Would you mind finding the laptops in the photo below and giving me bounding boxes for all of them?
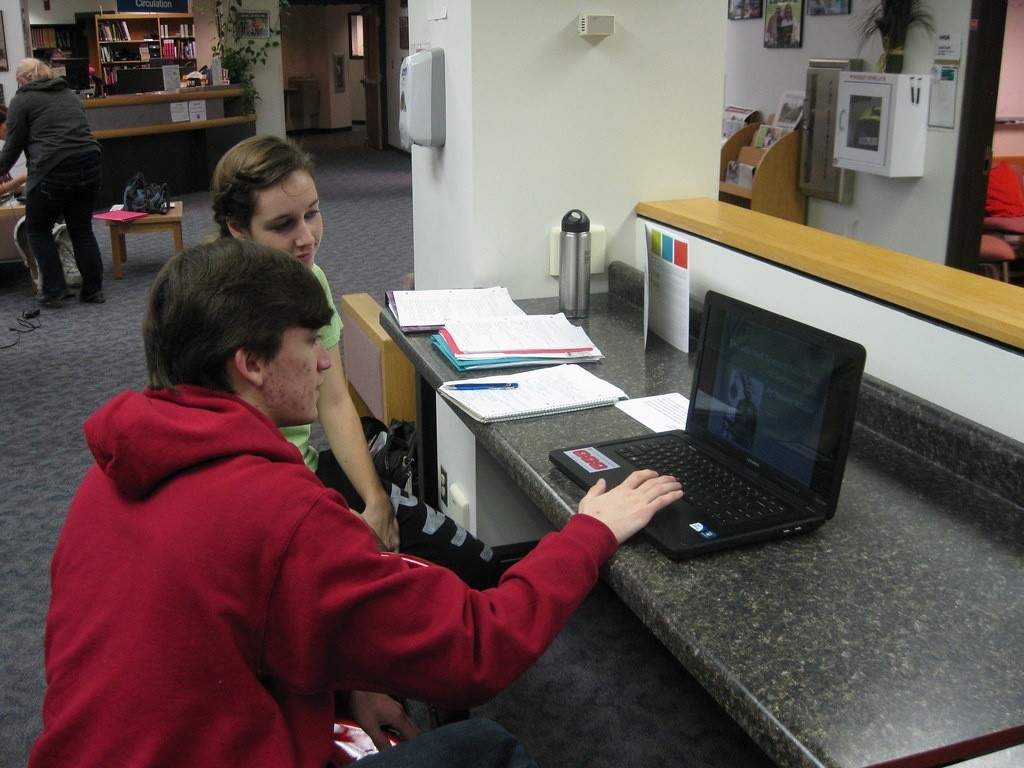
[550,289,867,562]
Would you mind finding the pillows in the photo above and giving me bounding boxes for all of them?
[985,163,1024,218]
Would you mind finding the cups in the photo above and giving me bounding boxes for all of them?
[558,209,589,318]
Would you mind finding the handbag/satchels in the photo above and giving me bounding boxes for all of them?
[124,174,169,215]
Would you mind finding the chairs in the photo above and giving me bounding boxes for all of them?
[978,232,1016,283]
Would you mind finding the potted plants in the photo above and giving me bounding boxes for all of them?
[194,0,291,117]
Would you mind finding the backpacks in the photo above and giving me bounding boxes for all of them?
[360,416,418,493]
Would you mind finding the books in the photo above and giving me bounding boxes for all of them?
[384,286,630,424]
[101,46,151,85]
[160,25,196,59]
[98,20,132,41]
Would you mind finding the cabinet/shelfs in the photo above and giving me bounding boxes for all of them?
[74,11,195,97]
[719,117,805,226]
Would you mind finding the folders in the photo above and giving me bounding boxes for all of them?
[93,211,148,223]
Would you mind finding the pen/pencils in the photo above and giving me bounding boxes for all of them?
[441,383,518,391]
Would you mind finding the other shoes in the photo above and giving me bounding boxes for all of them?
[78,289,105,303]
[36,282,68,302]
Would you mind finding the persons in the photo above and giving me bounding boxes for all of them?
[26,238,683,768]
[45,49,65,69]
[0,57,106,306]
[0,104,28,197]
[210,133,501,729]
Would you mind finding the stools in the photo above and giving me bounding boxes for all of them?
[105,201,183,280]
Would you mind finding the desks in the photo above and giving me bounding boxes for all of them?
[379,312,1024,768]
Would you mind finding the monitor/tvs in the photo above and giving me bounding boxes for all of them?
[114,68,166,94]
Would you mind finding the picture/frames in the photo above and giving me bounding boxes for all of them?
[233,10,269,41]
[728,0,760,19]
[807,0,851,16]
[763,0,803,50]
